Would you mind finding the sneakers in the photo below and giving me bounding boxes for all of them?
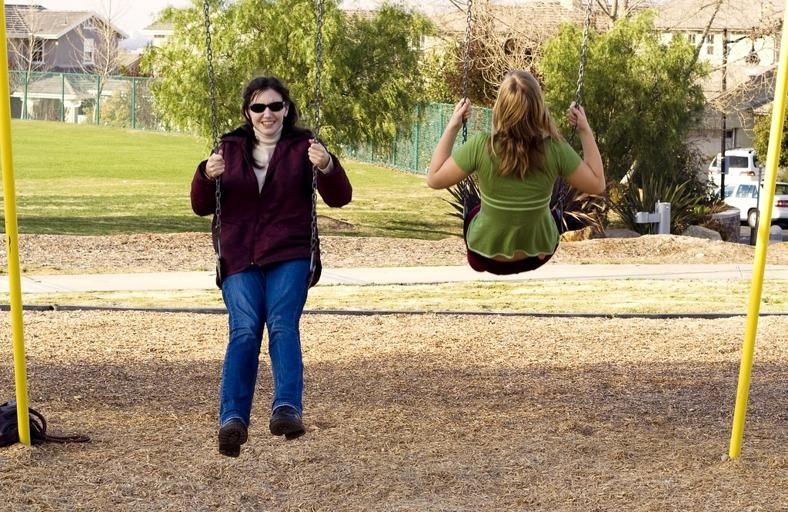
[269,406,306,440]
[218,421,247,457]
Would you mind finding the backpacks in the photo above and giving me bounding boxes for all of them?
[0,400,47,446]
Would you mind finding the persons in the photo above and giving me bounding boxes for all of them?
[427,68,606,275]
[191,78,353,458]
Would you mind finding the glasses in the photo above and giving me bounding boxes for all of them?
[249,102,283,113]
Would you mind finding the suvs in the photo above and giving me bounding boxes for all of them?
[709,181,788,230]
[708,145,785,194]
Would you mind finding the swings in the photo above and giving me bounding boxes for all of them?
[462,1,593,274]
[204,1,323,288]
